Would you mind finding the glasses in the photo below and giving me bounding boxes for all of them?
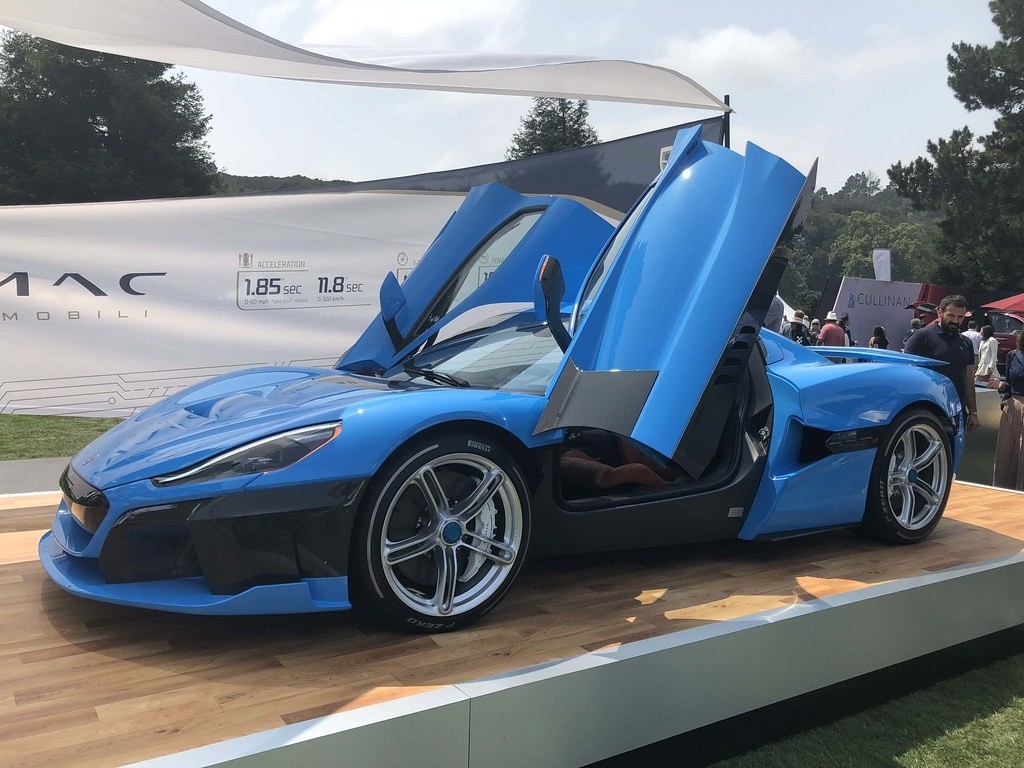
[812,323,819,325]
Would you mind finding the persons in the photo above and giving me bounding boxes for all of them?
[763,294,785,334]
[987,327,1024,491]
[961,319,1001,382]
[869,325,891,349]
[902,313,926,348]
[904,294,980,431]
[782,310,858,364]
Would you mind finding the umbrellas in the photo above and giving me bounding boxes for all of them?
[979,293,1024,324]
[904,298,938,314]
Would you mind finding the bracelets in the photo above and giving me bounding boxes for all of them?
[969,412,977,416]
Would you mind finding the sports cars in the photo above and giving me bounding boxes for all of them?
[38,123,965,634]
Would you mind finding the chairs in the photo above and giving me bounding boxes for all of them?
[562,435,681,495]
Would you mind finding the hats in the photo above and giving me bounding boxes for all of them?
[824,311,838,320]
